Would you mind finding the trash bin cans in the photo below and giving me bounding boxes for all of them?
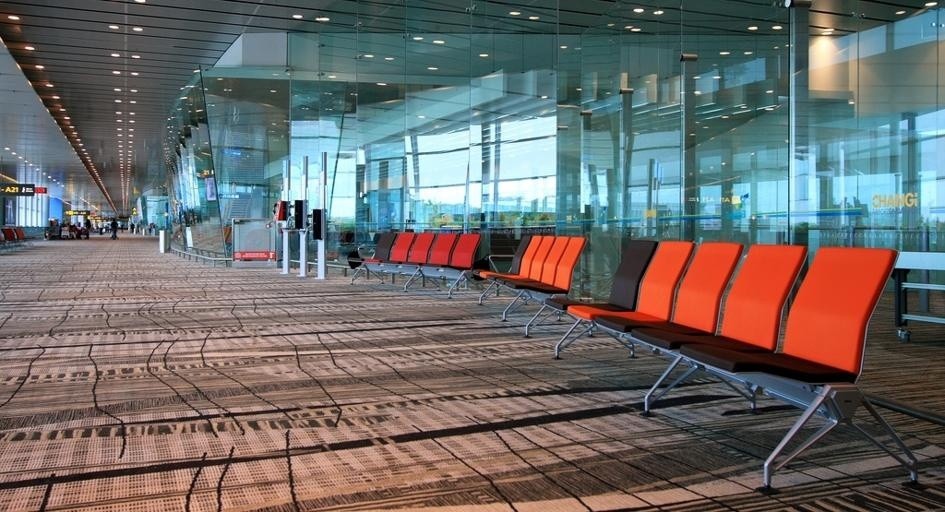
[159,230,170,253]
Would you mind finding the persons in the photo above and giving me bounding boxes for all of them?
[83,219,91,240]
[97,220,104,235]
[130,222,134,233]
[104,223,109,233]
[69,225,82,240]
[110,218,118,240]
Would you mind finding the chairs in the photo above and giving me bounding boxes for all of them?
[347,228,482,299]
